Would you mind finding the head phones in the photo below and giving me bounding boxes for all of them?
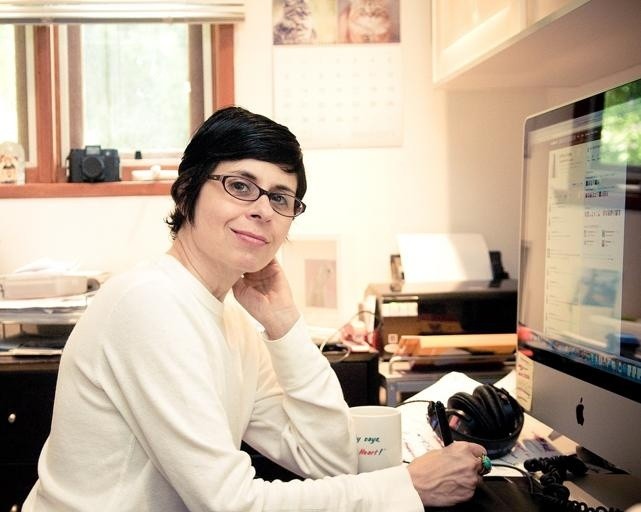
[428,381,526,457]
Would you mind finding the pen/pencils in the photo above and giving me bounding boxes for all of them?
[437,401,453,448]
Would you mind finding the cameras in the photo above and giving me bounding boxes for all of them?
[69,145,120,181]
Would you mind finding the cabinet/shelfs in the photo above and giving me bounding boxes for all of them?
[429,1,641,89]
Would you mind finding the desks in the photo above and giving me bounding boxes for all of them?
[377,361,516,407]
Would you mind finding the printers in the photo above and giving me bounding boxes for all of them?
[362,226,522,368]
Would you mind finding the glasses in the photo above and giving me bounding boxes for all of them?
[203,172,305,217]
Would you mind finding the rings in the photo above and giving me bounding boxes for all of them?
[478,454,492,476]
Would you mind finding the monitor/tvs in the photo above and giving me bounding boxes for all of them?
[515,76,641,512]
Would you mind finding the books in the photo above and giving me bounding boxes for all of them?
[0,262,113,361]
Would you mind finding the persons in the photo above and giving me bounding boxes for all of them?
[22,107,492,511]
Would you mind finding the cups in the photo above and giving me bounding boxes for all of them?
[349,405,402,474]
